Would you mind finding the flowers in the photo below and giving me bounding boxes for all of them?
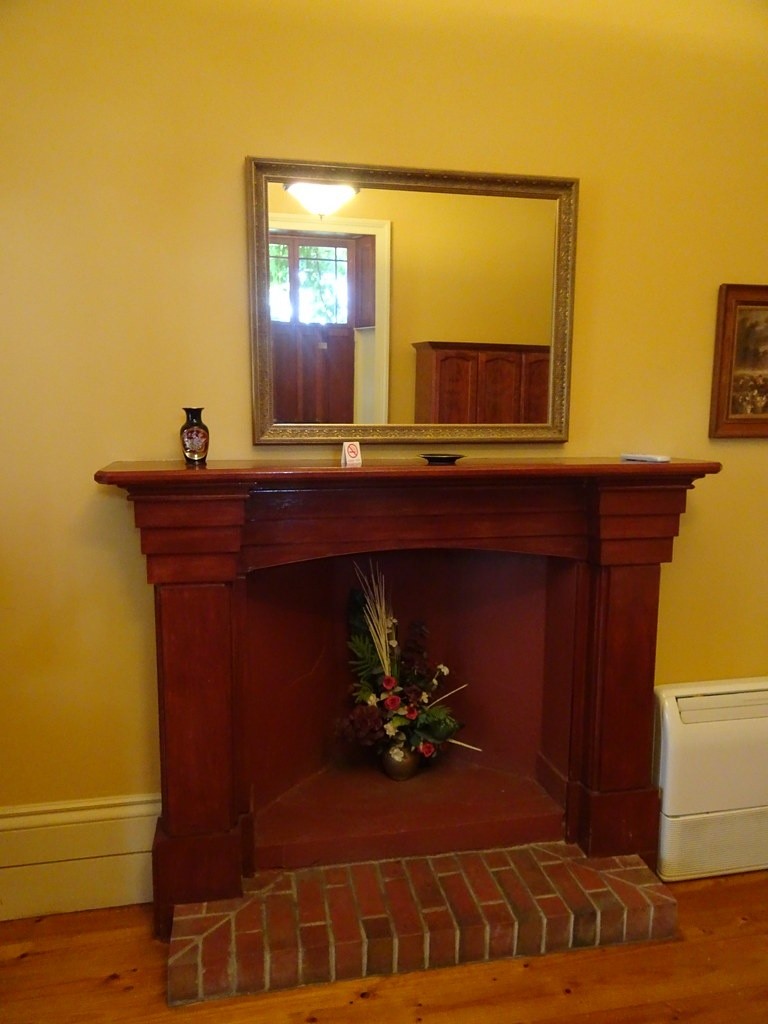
[339,558,484,759]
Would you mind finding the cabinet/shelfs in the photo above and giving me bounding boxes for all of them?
[410,341,550,425]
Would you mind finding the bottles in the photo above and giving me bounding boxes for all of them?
[178,407,209,466]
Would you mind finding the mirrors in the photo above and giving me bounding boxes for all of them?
[242,156,581,447]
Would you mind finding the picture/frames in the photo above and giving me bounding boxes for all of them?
[709,283,768,440]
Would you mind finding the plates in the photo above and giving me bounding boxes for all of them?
[417,453,465,464]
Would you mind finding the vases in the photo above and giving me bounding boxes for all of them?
[179,406,210,465]
[384,745,420,780]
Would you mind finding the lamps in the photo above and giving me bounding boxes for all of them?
[281,180,360,221]
